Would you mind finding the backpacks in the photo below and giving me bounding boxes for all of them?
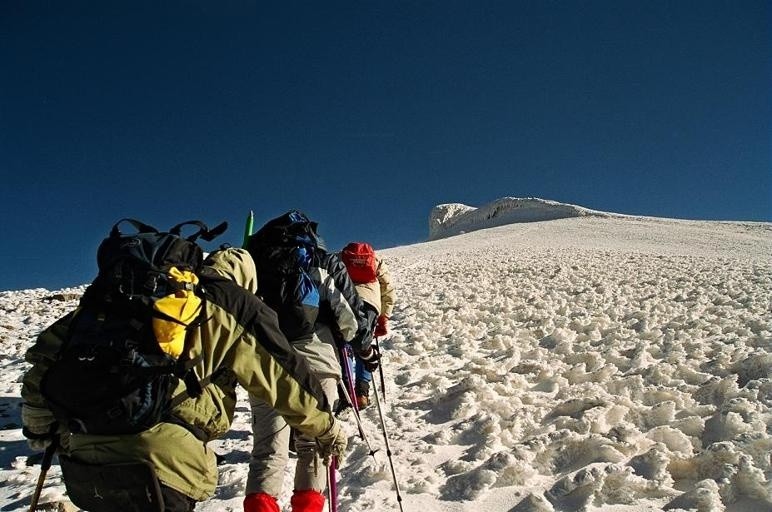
[247,208,321,337]
[39,232,204,434]
[340,241,378,282]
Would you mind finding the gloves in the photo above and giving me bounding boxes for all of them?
[358,347,381,372]
[22,403,56,453]
[316,418,347,470]
[374,316,389,336]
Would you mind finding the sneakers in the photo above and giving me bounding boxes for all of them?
[356,395,372,411]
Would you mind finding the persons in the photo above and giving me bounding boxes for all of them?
[335,242,398,409]
[20,247,350,511]
[243,210,374,512]
